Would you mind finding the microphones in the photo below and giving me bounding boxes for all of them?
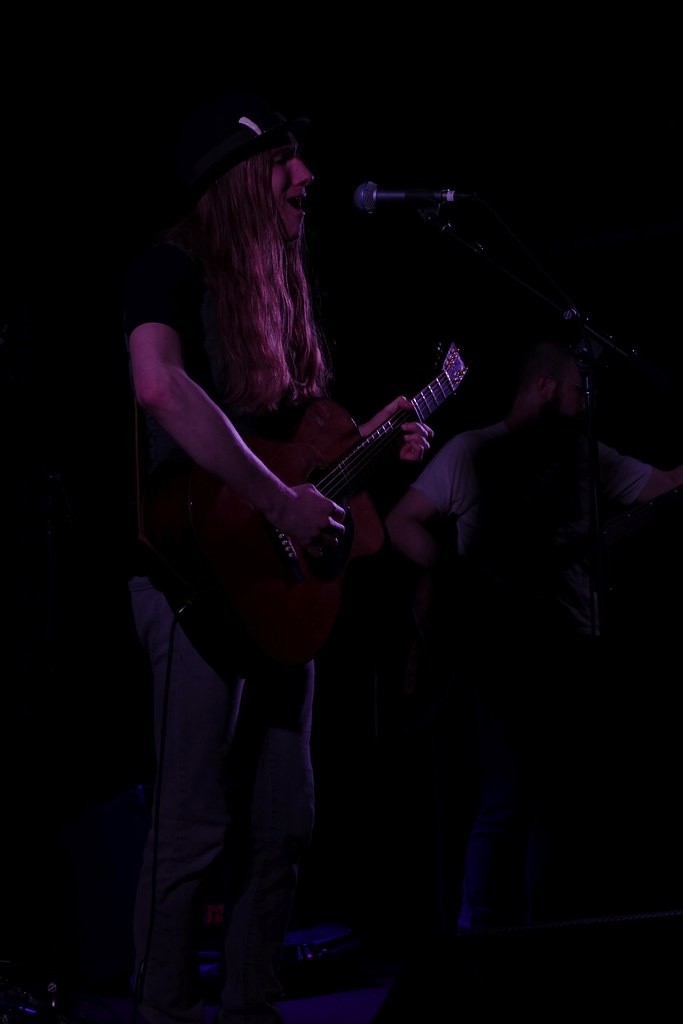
[354,181,457,213]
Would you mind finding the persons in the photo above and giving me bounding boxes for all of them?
[385,342,682,934]
[123,93,435,1024]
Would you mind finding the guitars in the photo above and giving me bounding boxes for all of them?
[149,321,466,672]
[532,482,683,638]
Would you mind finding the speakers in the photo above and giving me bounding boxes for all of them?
[426,910,683,1024]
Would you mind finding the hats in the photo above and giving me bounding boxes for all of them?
[193,109,310,200]
